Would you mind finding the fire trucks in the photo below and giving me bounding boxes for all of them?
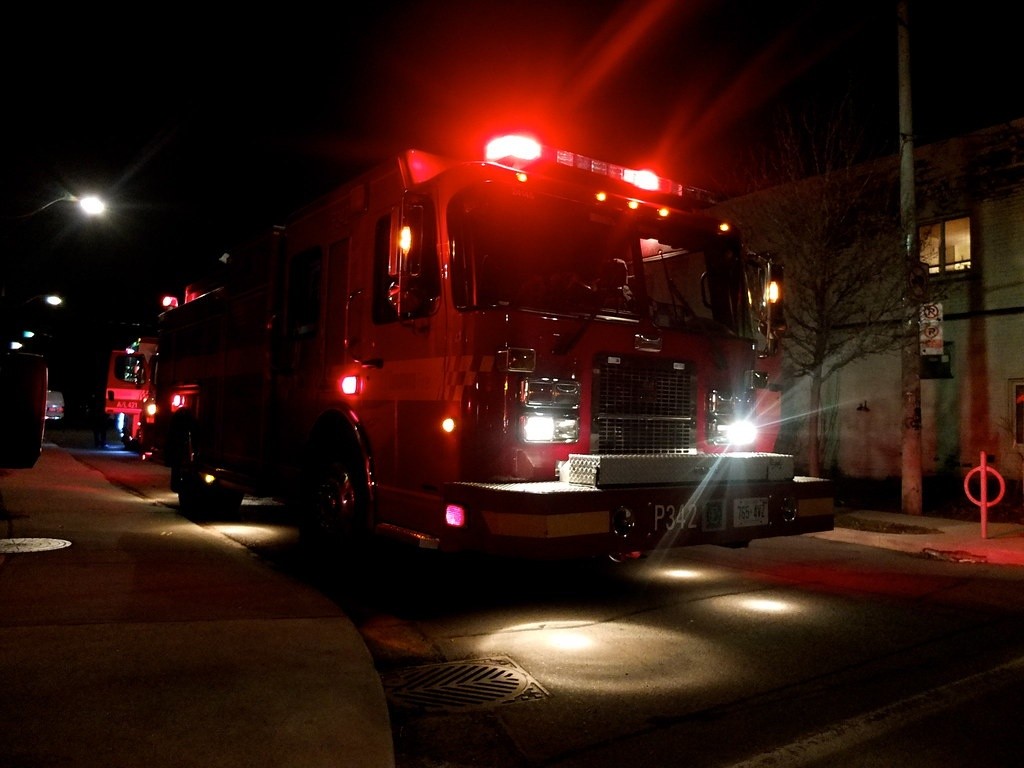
[103,132,835,611]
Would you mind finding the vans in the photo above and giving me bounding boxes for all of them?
[45,390,64,422]
[0,351,48,466]
